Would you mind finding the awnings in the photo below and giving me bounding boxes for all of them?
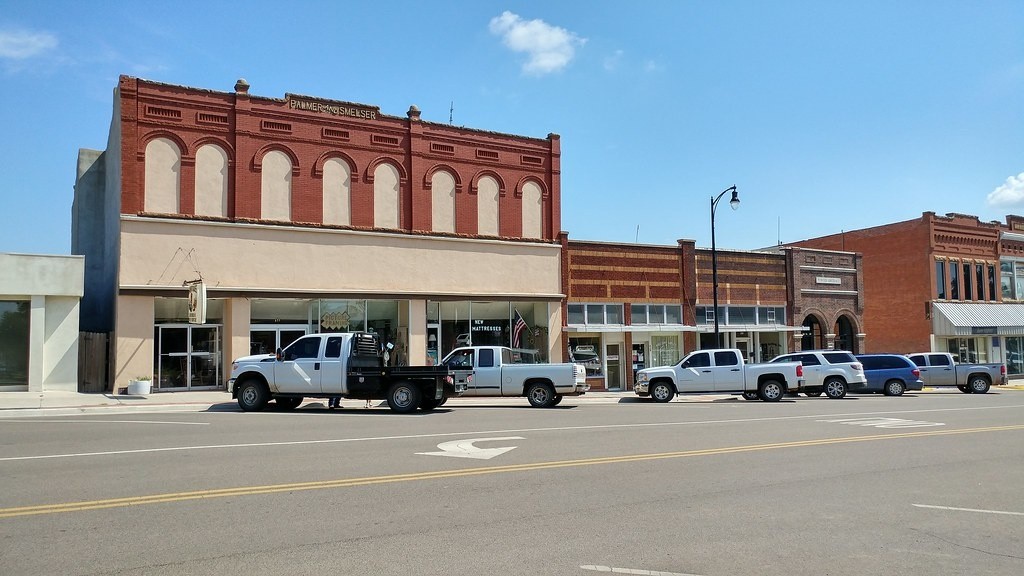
[697,323,810,333]
[933,302,1024,327]
[563,323,707,333]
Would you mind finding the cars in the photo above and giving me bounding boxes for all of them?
[572,344,602,375]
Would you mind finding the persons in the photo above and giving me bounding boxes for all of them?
[364,400,373,408]
[328,398,344,410]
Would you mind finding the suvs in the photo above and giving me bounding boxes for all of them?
[731,348,868,401]
[830,354,924,396]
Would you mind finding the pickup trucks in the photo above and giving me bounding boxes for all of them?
[225,331,475,414]
[897,352,1010,394]
[632,348,806,403]
[435,345,592,409]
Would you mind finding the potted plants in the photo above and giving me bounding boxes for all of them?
[127,376,152,395]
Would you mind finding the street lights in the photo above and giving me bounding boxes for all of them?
[710,184,739,349]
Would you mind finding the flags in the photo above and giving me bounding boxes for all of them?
[513,307,527,359]
